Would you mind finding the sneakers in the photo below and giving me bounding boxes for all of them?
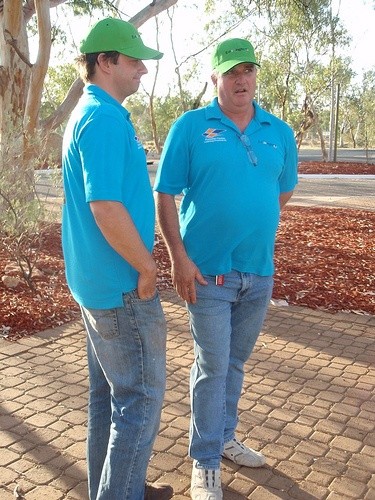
[220,439,266,467]
[190,458,223,500]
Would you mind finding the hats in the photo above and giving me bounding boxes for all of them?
[80,16,164,61]
[211,38,260,76]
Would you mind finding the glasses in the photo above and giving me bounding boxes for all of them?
[237,133,258,167]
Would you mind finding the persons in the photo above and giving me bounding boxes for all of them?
[153,38,299,500]
[61,15,174,500]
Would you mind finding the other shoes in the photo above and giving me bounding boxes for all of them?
[144,479,175,500]
